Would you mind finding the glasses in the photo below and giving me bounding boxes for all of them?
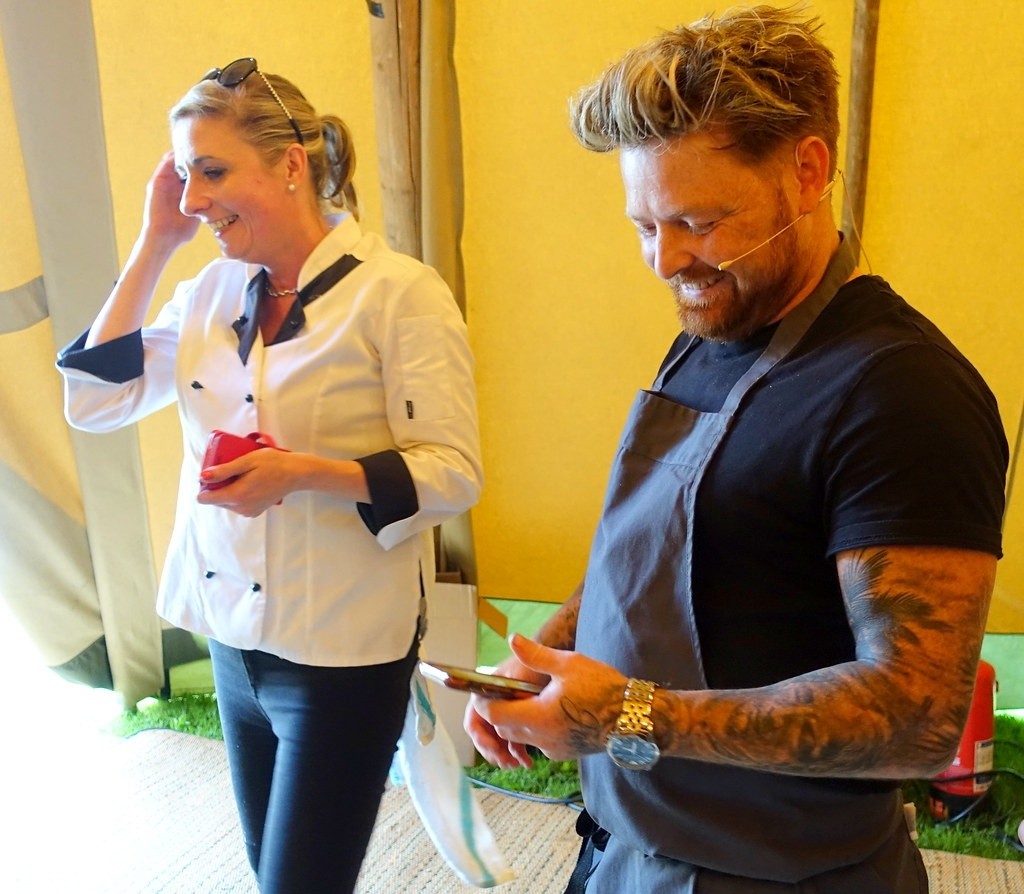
[200,57,304,148]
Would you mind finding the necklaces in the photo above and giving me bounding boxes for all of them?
[264,280,298,297]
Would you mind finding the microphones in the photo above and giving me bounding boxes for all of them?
[717,188,833,271]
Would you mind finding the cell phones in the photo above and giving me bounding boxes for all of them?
[417,661,543,703]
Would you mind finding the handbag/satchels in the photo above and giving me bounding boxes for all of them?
[397,658,519,889]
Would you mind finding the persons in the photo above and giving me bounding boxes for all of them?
[54,57,487,894]
[462,2,1010,894]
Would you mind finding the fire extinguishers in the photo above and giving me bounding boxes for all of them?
[927,659,999,821]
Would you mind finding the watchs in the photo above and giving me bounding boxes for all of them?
[604,677,661,772]
